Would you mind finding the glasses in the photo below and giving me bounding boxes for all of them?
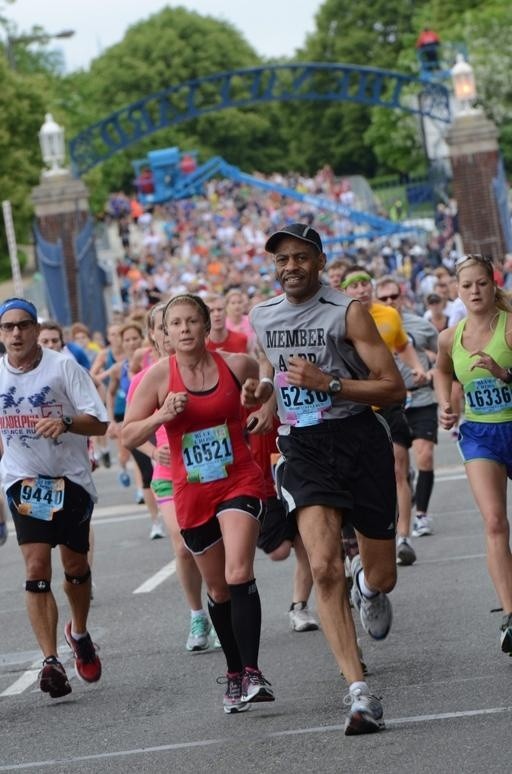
[454,253,490,267]
[378,294,399,302]
[1,320,35,331]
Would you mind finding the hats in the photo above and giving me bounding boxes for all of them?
[265,223,323,256]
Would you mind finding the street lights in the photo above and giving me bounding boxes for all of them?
[9,30,75,71]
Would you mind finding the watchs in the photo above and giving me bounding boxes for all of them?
[62,415,73,432]
[506,367,512,380]
[328,374,341,395]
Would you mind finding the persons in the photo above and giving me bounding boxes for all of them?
[239,380,320,631]
[121,295,275,716]
[203,297,250,353]
[89,325,125,386]
[248,224,407,737]
[71,323,111,469]
[223,296,249,335]
[127,166,455,291]
[425,294,451,331]
[105,327,166,542]
[376,279,440,538]
[423,284,451,320]
[62,340,88,372]
[124,303,223,651]
[0,299,109,698]
[341,269,429,565]
[37,322,65,353]
[432,256,512,657]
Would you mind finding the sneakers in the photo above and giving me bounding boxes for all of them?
[349,554,393,640]
[37,656,73,699]
[186,613,211,651]
[237,666,275,703]
[499,614,512,659]
[396,536,416,567]
[407,467,415,510]
[63,620,102,684]
[339,692,386,737]
[135,489,145,504]
[215,672,251,714]
[149,524,165,540]
[213,632,222,652]
[411,514,431,537]
[290,607,319,633]
